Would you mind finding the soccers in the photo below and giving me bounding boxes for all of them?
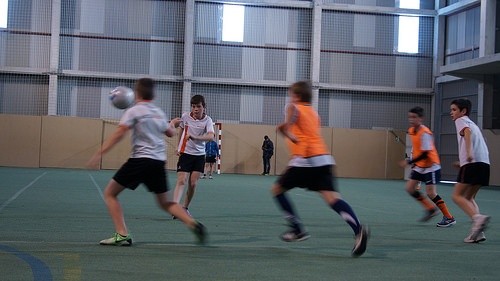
[109,86,135,109]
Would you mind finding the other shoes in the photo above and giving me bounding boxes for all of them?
[172,215,176,219]
[200,175,207,179]
[185,209,191,216]
[209,175,213,179]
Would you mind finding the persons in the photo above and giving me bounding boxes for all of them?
[450,99,491,243]
[172,95,215,220]
[86,79,209,246]
[271,81,368,258]
[399,106,457,228]
[200,134,219,178]
[262,136,274,175]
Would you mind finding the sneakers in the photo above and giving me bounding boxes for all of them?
[417,206,441,222]
[351,225,368,257]
[469,214,491,240]
[192,220,206,243]
[100,233,132,247]
[464,230,486,242]
[279,230,311,242]
[437,216,456,227]
